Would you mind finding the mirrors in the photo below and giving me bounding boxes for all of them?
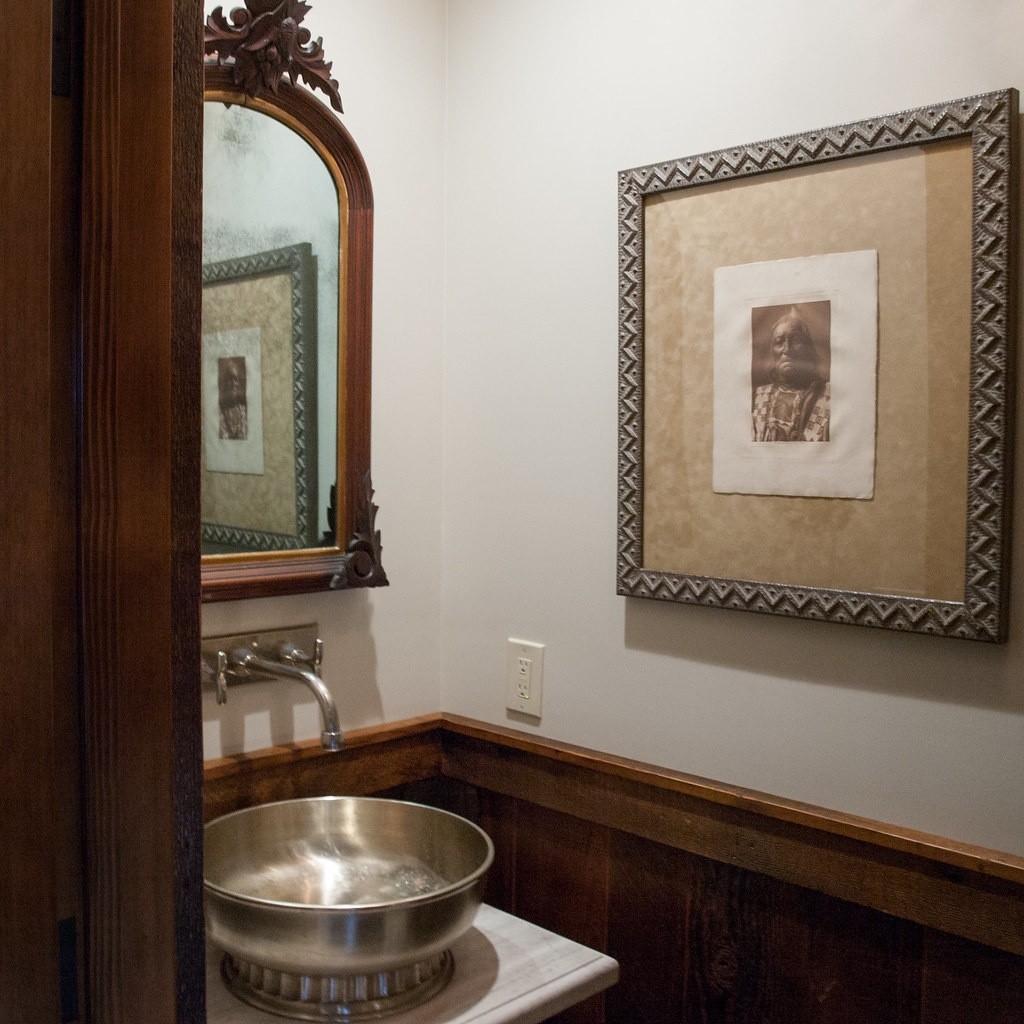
[201,1,392,602]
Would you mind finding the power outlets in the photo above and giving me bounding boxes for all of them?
[504,637,545,719]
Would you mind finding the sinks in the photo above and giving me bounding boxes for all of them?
[205,797,495,1024]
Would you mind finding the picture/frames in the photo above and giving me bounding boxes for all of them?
[614,85,1021,642]
[204,239,308,551]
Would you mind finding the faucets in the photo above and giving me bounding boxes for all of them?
[200,623,342,752]
